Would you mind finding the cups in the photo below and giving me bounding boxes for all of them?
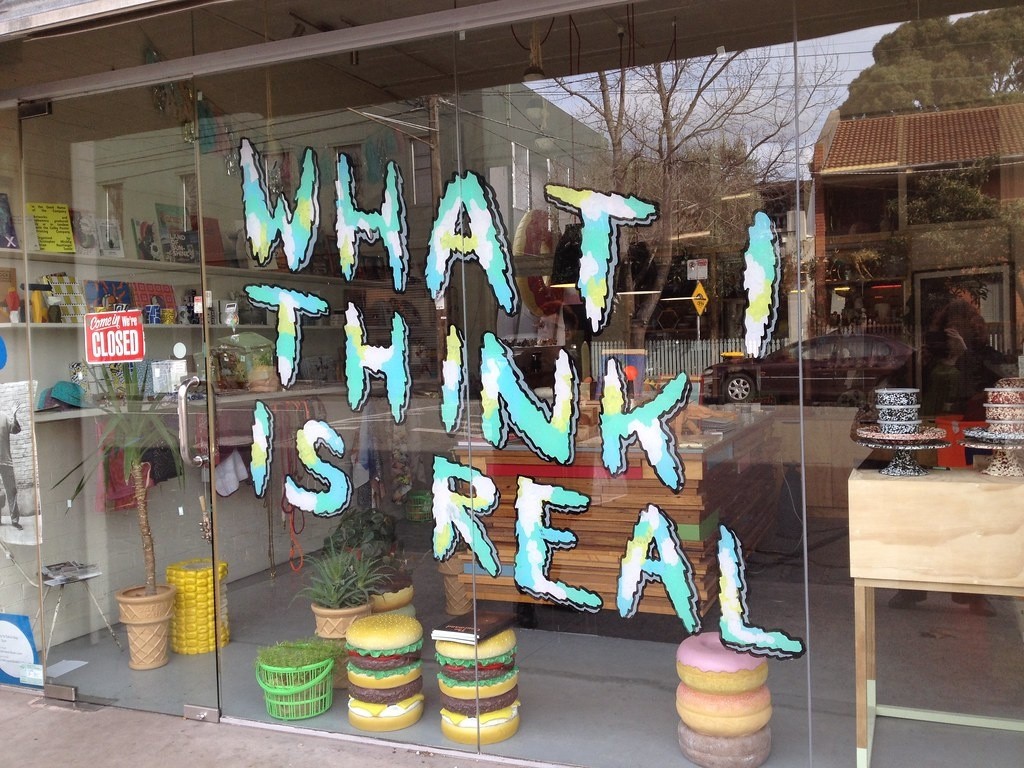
[113,303,128,311]
[162,309,175,324]
[145,305,159,324]
[178,305,187,318]
[94,307,106,312]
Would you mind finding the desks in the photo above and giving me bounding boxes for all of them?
[847,449,1024,768]
[448,407,778,645]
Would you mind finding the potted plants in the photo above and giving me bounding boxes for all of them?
[287,530,397,689]
[48,359,196,671]
[255,638,347,723]
[323,505,401,566]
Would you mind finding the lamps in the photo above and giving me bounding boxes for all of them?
[550,223,701,301]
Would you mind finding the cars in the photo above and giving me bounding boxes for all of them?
[701,334,918,404]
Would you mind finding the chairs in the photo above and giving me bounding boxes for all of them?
[0,538,125,662]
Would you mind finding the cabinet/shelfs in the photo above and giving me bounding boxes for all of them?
[0,246,443,423]
[751,405,952,519]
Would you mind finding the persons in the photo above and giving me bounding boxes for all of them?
[890,300,1007,618]
